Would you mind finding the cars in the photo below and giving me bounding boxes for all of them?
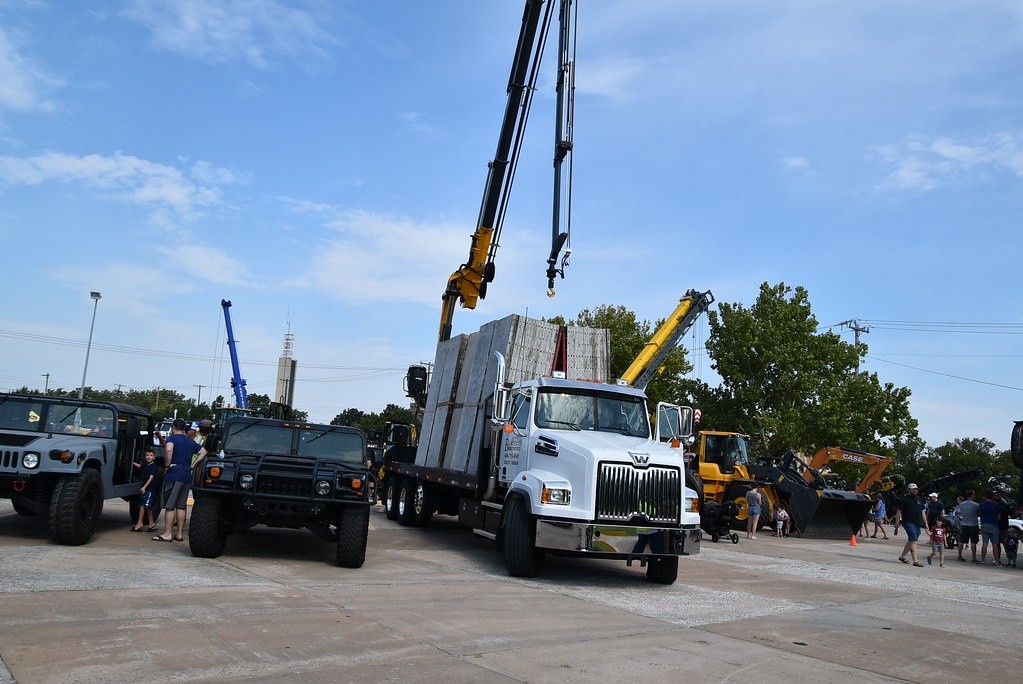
[153,423,172,438]
[977,512,1023,539]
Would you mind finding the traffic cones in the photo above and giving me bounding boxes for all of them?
[849,534,858,546]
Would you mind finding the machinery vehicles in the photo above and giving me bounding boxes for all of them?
[211,407,255,429]
[208,299,248,410]
[685,431,892,540]
[367,1,578,503]
[71,417,127,436]
[619,289,715,424]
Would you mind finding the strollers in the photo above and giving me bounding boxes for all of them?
[941,515,968,549]
[700,501,744,545]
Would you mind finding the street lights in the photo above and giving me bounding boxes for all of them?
[74,292,102,433]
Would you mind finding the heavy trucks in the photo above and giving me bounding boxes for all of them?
[382,350,702,585]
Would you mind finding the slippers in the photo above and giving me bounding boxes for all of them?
[151,535,172,542]
[173,535,183,541]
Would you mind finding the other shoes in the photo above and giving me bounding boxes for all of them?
[971,560,979,564]
[130,526,143,532]
[940,564,945,568]
[927,557,932,565]
[957,558,966,562]
[144,525,159,532]
[752,536,758,539]
[980,562,985,565]
[994,562,1004,567]
[913,562,924,567]
[870,535,877,538]
[881,537,889,540]
[1005,564,1016,568]
[747,534,751,538]
[899,557,910,564]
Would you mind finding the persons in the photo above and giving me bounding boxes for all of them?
[870,494,890,539]
[745,482,761,540]
[859,488,872,539]
[892,482,1019,569]
[773,505,791,538]
[132,418,215,542]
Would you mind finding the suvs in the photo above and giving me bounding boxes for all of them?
[189,416,384,568]
[0,393,166,546]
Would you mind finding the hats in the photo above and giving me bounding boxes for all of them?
[908,483,918,489]
[196,420,213,428]
[170,419,186,430]
[929,492,938,498]
